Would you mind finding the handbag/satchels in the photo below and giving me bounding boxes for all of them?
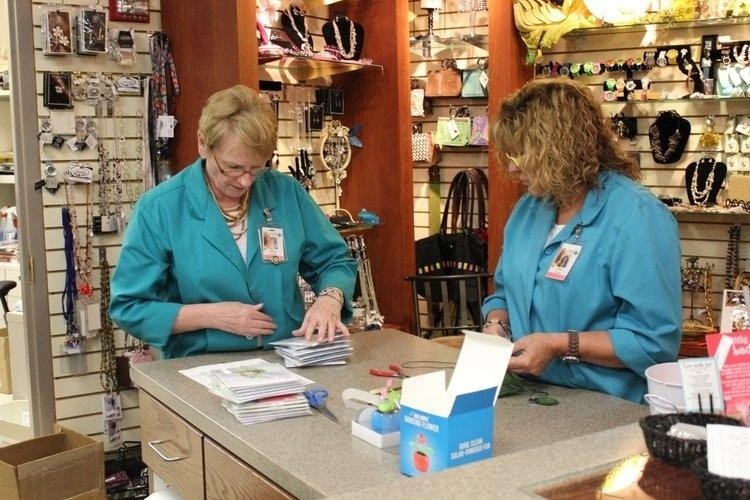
[415,168,489,304]
[410,59,489,168]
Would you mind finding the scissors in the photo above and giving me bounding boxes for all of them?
[303,389,339,428]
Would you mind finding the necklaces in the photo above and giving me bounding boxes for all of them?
[689,160,717,203]
[210,184,251,239]
[331,19,358,57]
[50,100,145,443]
[648,122,681,163]
[285,3,313,52]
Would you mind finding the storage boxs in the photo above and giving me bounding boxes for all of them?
[0,421,108,500]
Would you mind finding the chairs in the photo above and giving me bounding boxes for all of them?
[402,270,494,348]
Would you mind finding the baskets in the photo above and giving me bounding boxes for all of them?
[639,411,750,500]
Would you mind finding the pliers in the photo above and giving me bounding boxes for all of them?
[369,364,412,380]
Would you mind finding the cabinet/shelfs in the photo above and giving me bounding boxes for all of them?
[140,389,294,499]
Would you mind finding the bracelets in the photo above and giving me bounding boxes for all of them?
[483,317,512,338]
[319,287,345,306]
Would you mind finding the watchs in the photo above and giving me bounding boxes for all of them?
[560,329,581,367]
[601,79,638,101]
[540,47,688,78]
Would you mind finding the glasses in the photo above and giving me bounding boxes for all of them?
[208,145,273,177]
[505,152,530,171]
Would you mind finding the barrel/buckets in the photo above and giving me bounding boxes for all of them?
[644,361,688,416]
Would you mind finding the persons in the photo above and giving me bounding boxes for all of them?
[476,78,681,402]
[109,83,362,361]
